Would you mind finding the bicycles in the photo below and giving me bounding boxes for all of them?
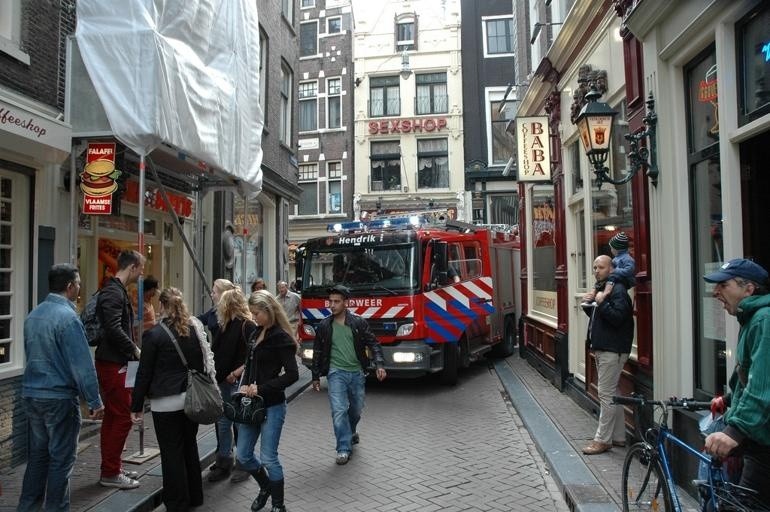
[605,395,769,512]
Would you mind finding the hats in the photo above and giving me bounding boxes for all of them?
[703,258,769,284]
[327,285,351,297]
[609,232,629,249]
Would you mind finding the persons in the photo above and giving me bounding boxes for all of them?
[581,255,634,455]
[195,278,301,483]
[95,250,143,489]
[580,231,636,309]
[130,286,209,511]
[312,284,387,465]
[448,263,461,283]
[235,289,297,511]
[130,276,159,414]
[702,259,770,512]
[17,263,106,512]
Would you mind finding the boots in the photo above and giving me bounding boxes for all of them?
[208,452,234,482]
[231,457,249,483]
[268,477,286,512]
[249,466,270,512]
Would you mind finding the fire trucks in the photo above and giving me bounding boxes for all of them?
[290,207,523,387]
[593,206,636,273]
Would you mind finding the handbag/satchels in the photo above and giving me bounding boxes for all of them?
[222,392,265,424]
[184,368,224,424]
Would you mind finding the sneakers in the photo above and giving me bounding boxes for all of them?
[335,448,353,465]
[611,440,625,446]
[120,468,138,478]
[351,433,359,444]
[100,474,140,489]
[582,441,611,454]
[591,302,598,306]
[580,301,591,307]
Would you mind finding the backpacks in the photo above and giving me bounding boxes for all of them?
[81,289,101,347]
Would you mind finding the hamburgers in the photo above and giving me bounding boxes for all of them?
[79,159,122,197]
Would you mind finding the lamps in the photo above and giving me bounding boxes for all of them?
[572,82,658,192]
